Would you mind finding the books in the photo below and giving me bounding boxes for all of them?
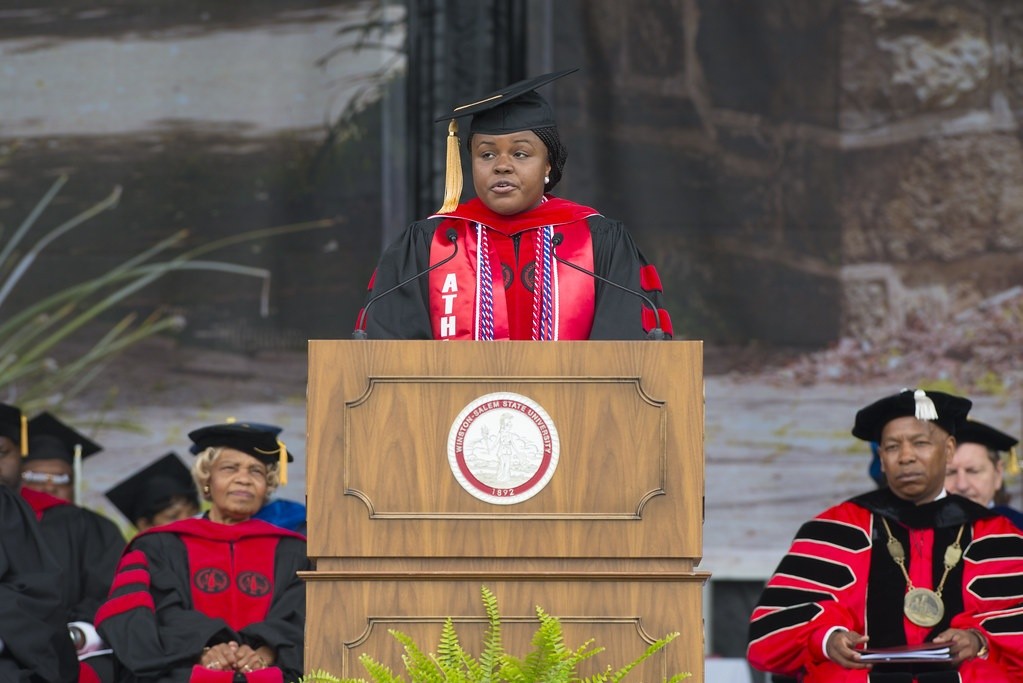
[856,641,960,664]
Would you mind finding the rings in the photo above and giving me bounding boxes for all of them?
[206,663,213,668]
[244,664,250,671]
[214,661,219,666]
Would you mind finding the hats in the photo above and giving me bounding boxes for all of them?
[0,403,28,457]
[435,67,580,215]
[18,412,104,466]
[851,387,973,448]
[188,420,293,488]
[104,452,201,528]
[955,419,1019,476]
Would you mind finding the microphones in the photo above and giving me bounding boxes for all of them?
[353,227,458,340]
[551,231,666,340]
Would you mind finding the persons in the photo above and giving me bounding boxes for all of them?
[354,67,672,340]
[746,390,1023,683]
[0,403,308,683]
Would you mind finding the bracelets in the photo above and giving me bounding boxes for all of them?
[970,629,988,656]
[257,656,267,667]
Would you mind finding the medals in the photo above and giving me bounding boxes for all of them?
[905,587,945,627]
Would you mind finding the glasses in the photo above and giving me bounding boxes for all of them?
[21,470,74,488]
